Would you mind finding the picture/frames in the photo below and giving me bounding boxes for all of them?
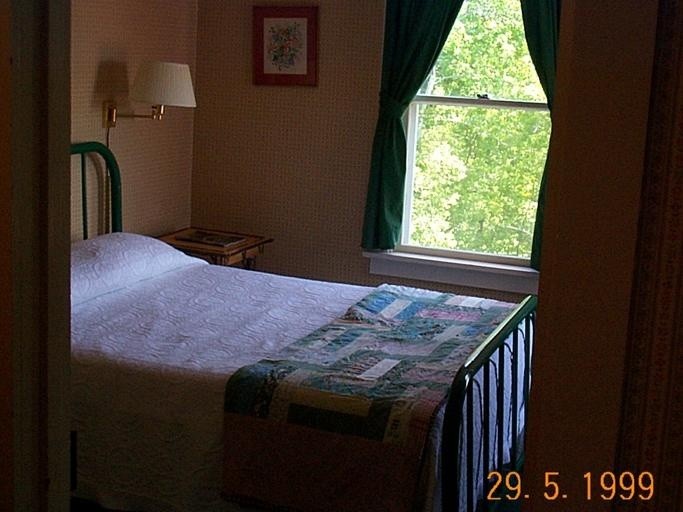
[250,1,322,89]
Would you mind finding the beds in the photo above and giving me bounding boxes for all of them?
[70,140,538,506]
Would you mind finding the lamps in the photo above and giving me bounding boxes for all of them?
[103,58,201,134]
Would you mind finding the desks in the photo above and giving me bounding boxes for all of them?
[155,222,276,272]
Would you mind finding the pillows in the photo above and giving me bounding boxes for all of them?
[69,230,208,308]
